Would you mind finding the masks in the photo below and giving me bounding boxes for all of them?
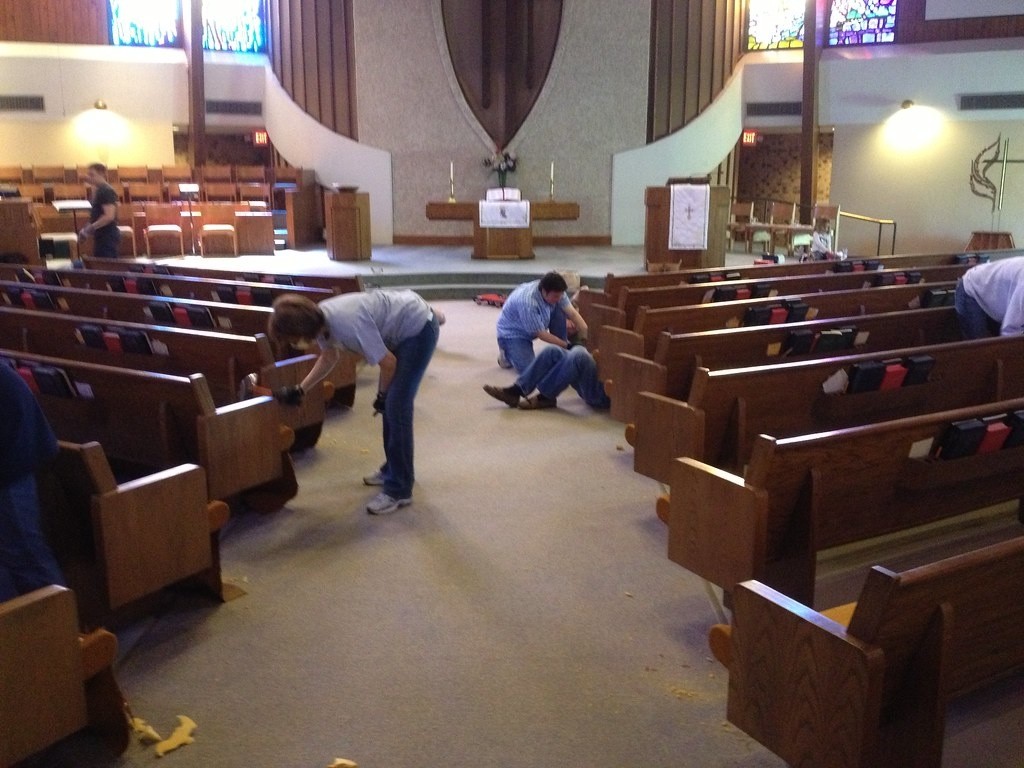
[291,334,315,350]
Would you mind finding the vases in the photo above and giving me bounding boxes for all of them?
[498,170,507,187]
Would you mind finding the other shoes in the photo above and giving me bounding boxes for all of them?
[482,384,520,407]
[518,393,557,409]
[498,347,513,368]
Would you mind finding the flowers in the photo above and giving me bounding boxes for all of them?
[481,149,518,177]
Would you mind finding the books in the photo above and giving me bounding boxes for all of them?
[690,272,743,282]
[822,354,937,392]
[936,409,1024,458]
[921,289,955,309]
[834,261,881,271]
[9,265,294,400]
[746,297,809,325]
[713,284,779,301]
[789,326,859,352]
[955,254,989,266]
[878,272,921,285]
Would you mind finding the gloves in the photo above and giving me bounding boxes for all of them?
[78,223,94,245]
[271,384,304,406]
[374,391,387,413]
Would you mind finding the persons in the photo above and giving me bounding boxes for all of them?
[78,164,120,258]
[954,256,1024,338]
[495,272,588,374]
[811,217,832,261]
[482,345,610,409]
[0,358,64,601]
[268,286,446,515]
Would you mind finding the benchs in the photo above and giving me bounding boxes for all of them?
[0,256,366,768]
[578,250,1024,768]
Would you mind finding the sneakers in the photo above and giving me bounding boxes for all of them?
[366,493,412,514]
[363,468,386,485]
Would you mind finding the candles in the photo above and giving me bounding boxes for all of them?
[450,160,454,179]
[550,160,554,182]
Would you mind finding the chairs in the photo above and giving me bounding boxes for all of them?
[725,200,841,258]
[0,166,325,263]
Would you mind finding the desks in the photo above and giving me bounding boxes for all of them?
[425,202,581,261]
[234,212,275,256]
